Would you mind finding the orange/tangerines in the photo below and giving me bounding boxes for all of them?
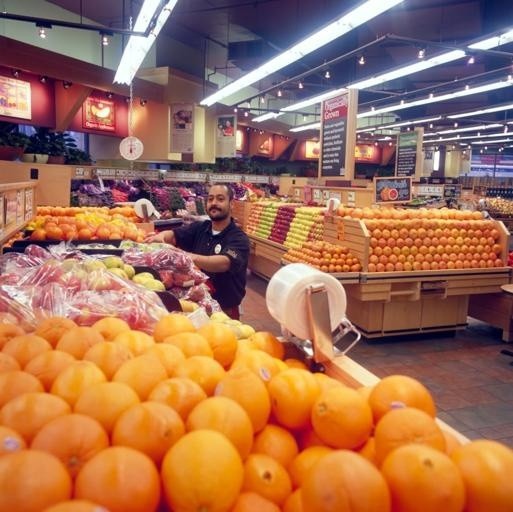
[0,312,513,512]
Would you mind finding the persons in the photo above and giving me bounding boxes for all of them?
[146,182,249,320]
[128,178,149,202]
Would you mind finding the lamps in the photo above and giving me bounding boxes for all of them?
[199,1,401,108]
[39,28,47,39]
[112,0,177,85]
[252,28,512,154]
[103,37,109,46]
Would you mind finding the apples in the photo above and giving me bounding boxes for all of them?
[0,240,200,335]
[253,203,326,249]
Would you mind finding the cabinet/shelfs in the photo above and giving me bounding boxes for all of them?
[275,336,471,452]
[231,187,372,283]
[467,209,513,340]
[324,200,512,342]
